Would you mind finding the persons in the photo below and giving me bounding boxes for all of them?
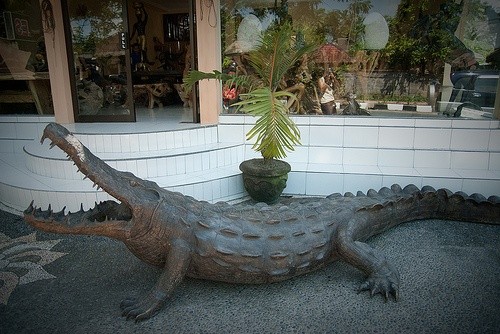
[316,62,339,115]
[130,43,151,73]
[129,0,148,56]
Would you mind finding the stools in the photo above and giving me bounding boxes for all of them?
[0,72,50,114]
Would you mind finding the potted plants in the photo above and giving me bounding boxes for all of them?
[183,19,321,203]
